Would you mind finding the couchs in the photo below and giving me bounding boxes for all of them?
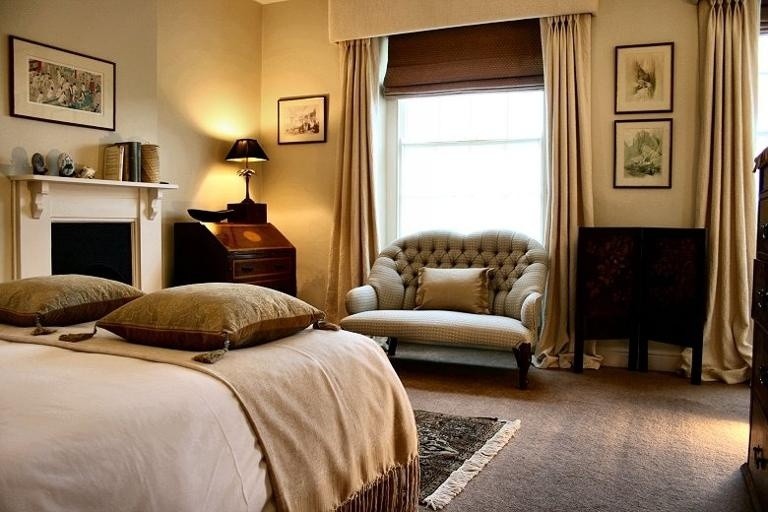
[338,228,547,390]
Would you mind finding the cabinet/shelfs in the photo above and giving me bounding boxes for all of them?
[170,220,298,298]
[738,145,767,512]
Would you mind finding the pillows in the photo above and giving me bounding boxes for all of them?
[57,283,328,363]
[414,266,494,315]
[0,273,144,336]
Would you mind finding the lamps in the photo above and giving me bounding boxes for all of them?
[224,137,269,225]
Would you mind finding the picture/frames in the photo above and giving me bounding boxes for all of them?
[612,40,674,114]
[610,117,674,190]
[275,93,327,145]
[6,32,116,132]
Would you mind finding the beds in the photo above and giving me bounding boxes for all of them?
[0,289,420,511]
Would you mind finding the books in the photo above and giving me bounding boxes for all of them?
[104,142,142,182]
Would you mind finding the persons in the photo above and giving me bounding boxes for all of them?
[32,68,96,105]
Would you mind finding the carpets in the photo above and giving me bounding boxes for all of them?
[411,405,522,511]
